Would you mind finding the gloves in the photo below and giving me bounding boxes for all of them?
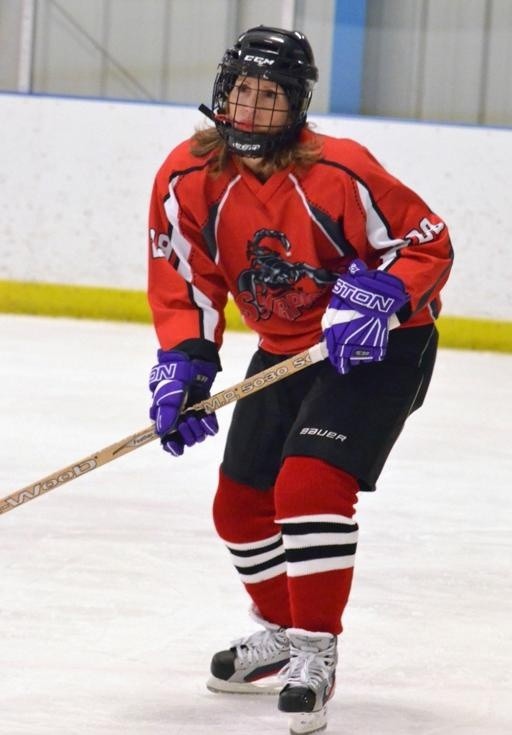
[319,257,411,375]
[147,336,223,458]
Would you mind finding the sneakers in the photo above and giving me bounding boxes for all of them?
[277,626,339,714]
[211,602,292,685]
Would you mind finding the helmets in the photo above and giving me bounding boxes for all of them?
[211,24,319,157]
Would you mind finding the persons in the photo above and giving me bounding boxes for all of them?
[150,29,454,714]
